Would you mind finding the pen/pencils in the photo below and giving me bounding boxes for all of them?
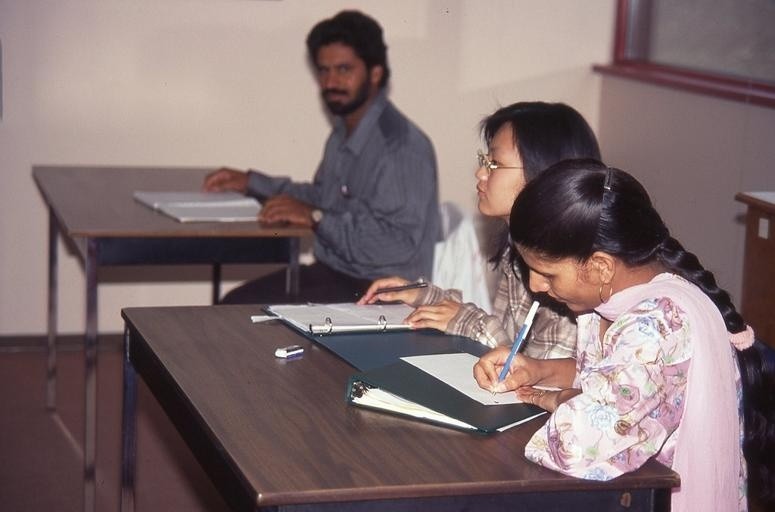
[492,300,540,396]
[352,283,428,297]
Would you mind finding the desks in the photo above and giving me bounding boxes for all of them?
[121,303,681,509]
[30,163,315,509]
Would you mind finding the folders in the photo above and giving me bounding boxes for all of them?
[260,302,562,433]
[345,376,494,437]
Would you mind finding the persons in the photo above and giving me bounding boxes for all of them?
[354,100,607,359]
[200,10,440,307]
[472,154,774,511]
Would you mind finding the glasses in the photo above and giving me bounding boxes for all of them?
[474,148,525,172]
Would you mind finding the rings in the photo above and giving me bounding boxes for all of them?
[529,394,538,405]
[543,389,551,394]
[537,390,546,397]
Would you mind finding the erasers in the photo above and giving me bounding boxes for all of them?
[275,345,304,358]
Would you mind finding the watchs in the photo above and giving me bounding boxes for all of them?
[309,206,326,233]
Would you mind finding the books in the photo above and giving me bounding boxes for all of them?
[341,350,551,435]
[257,298,493,374]
[133,187,268,224]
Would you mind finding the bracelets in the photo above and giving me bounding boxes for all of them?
[554,387,581,407]
